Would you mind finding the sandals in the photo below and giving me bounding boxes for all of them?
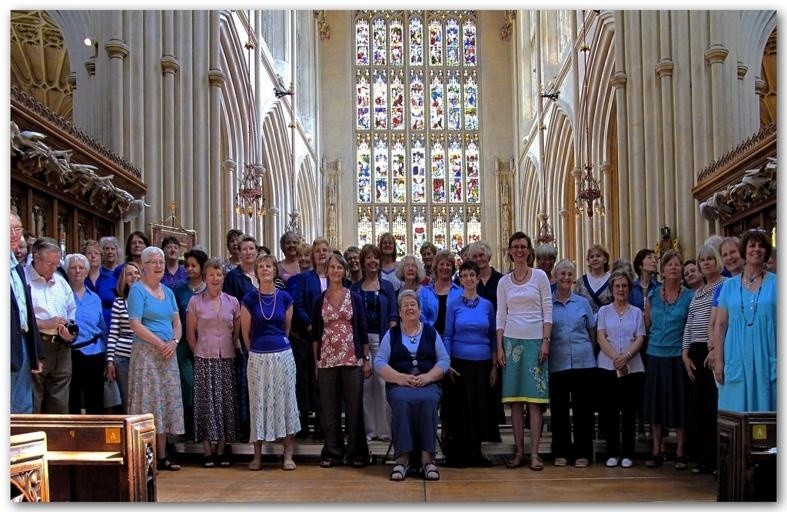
[645,456,666,468]
[530,454,544,471]
[422,461,440,480]
[675,455,687,470]
[319,458,335,468]
[351,457,368,468]
[506,452,524,468]
[390,462,407,480]
[157,457,181,470]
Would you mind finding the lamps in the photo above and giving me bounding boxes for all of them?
[538,217,557,250]
[285,211,301,237]
[577,163,605,219]
[82,34,100,59]
[235,165,267,220]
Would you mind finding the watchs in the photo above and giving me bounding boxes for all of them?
[363,355,370,361]
[543,336,551,342]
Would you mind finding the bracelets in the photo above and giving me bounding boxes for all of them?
[172,338,179,345]
[628,351,633,358]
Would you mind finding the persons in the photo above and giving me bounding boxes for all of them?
[682,259,706,290]
[23,237,76,414]
[350,245,399,442]
[222,229,243,277]
[701,236,745,370]
[526,247,534,267]
[763,246,776,273]
[550,259,596,467]
[343,246,364,282]
[102,237,122,278]
[597,272,646,468]
[11,211,46,414]
[63,253,108,414]
[159,237,190,288]
[128,246,185,470]
[13,234,27,266]
[24,231,37,252]
[125,231,150,266]
[285,242,313,293]
[379,233,405,290]
[573,244,615,440]
[681,244,729,476]
[107,262,142,415]
[496,231,553,470]
[631,249,663,441]
[193,244,208,256]
[241,255,302,471]
[420,242,436,286]
[703,235,731,278]
[416,251,463,336]
[645,250,694,469]
[536,243,558,292]
[441,261,497,467]
[277,231,302,283]
[311,255,372,467]
[395,254,440,326]
[222,234,258,443]
[461,247,471,262]
[174,249,208,442]
[613,259,636,280]
[258,246,271,259]
[80,239,117,327]
[290,237,331,440]
[184,257,241,467]
[713,228,777,413]
[455,241,504,312]
[374,289,450,480]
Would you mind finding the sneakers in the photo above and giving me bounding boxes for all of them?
[606,457,619,467]
[555,458,567,466]
[621,458,632,468]
[576,459,588,467]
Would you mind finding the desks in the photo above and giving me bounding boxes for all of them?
[717,409,776,501]
[11,414,156,503]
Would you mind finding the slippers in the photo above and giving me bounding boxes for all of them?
[217,456,231,468]
[203,455,215,468]
[282,458,297,470]
[249,459,262,471]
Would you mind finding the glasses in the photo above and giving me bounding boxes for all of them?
[11,226,25,234]
[42,260,61,268]
[144,258,165,265]
[460,273,478,279]
[747,228,767,232]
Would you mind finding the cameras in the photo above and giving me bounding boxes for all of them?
[57,320,80,339]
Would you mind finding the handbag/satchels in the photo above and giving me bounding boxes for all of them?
[103,379,122,408]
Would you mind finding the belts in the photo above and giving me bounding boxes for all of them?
[40,332,61,344]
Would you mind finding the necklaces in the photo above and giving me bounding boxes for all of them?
[513,269,529,282]
[463,297,480,308]
[740,271,763,327]
[743,272,762,282]
[663,285,681,315]
[150,287,165,302]
[614,303,629,315]
[365,281,376,317]
[401,323,421,343]
[701,280,721,295]
[258,289,276,321]
[189,281,203,292]
